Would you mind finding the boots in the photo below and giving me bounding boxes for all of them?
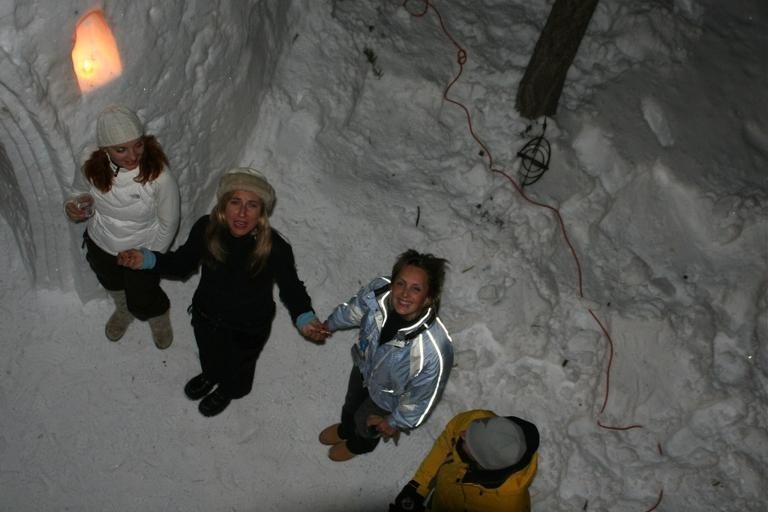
[147,308,173,349]
[105,288,134,342]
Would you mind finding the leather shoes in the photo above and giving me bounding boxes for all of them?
[199,386,231,417]
[185,374,214,400]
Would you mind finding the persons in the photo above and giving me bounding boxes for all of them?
[63,106,180,348]
[390,409,540,511]
[124,169,320,416]
[309,250,454,462]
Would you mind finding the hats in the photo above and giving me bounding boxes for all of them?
[466,416,527,471]
[97,105,143,148]
[218,168,277,217]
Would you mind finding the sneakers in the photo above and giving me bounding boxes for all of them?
[319,423,348,445]
[329,440,358,461]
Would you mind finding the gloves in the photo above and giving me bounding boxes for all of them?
[389,485,426,511]
[366,415,410,446]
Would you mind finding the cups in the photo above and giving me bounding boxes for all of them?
[74,193,95,218]
